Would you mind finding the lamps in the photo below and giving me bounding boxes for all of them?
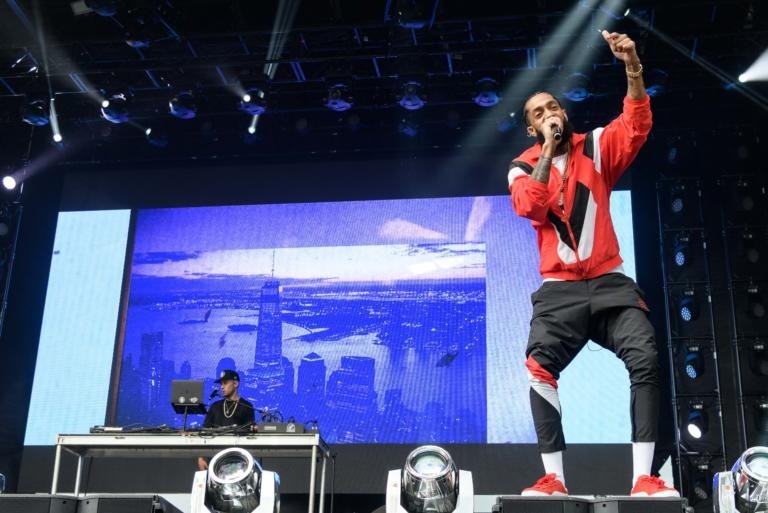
[713,445,768,513]
[1,0,767,125]
[656,144,768,441]
[191,446,280,513]
[385,445,475,513]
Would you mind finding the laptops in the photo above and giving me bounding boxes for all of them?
[171,380,208,415]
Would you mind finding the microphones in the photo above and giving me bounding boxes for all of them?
[209,389,218,400]
[552,125,563,140]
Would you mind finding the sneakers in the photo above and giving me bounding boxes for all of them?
[631,473,680,497]
[521,473,569,496]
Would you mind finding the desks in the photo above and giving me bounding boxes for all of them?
[52,434,331,513]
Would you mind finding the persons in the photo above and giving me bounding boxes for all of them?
[198,370,257,471]
[508,30,679,499]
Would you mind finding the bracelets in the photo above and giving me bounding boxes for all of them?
[627,63,644,79]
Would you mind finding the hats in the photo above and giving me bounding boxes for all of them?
[214,369,240,383]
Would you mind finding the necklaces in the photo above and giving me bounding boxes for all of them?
[562,139,569,180]
[224,395,239,418]
